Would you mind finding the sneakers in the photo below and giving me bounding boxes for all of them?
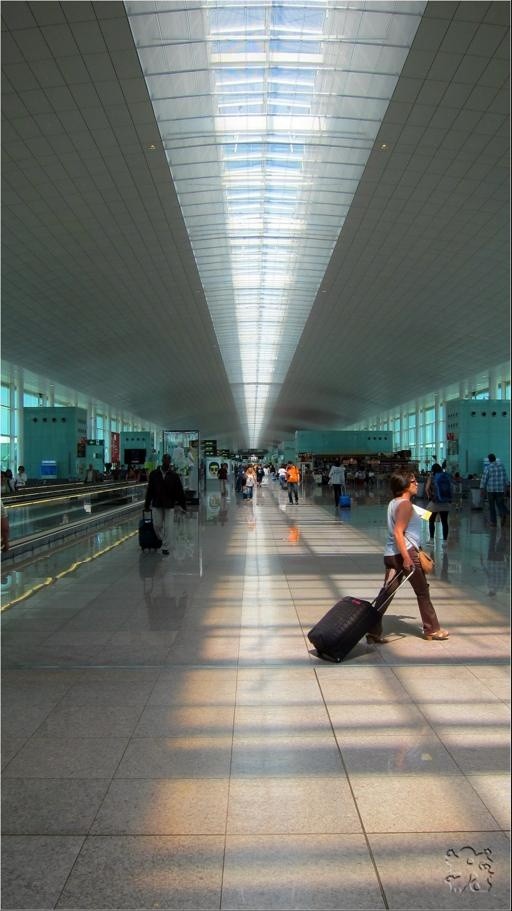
[441,539,448,546]
[426,536,436,545]
[160,548,170,555]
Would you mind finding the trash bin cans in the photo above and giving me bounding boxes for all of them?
[470,486,484,510]
[417,480,426,499]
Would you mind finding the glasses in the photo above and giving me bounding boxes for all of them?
[409,477,418,484]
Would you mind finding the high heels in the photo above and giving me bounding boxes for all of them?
[361,627,391,648]
[422,623,452,642]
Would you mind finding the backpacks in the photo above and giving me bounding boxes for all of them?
[430,472,455,505]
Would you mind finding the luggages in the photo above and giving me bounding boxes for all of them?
[137,507,163,553]
[339,484,352,510]
[303,561,418,665]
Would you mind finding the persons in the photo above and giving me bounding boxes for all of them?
[366,471,450,643]
[1,445,510,554]
